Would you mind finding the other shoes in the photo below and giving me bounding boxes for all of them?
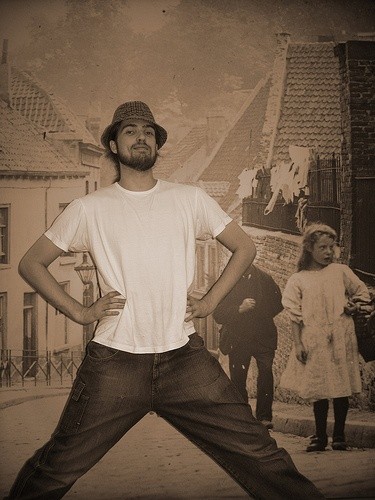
[259,417,273,428]
[331,440,347,451]
[307,436,328,451]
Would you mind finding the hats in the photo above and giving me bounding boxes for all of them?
[99,100,170,150]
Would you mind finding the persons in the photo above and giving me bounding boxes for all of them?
[213,263,285,429]
[280,223,374,452]
[3,102,325,500]
